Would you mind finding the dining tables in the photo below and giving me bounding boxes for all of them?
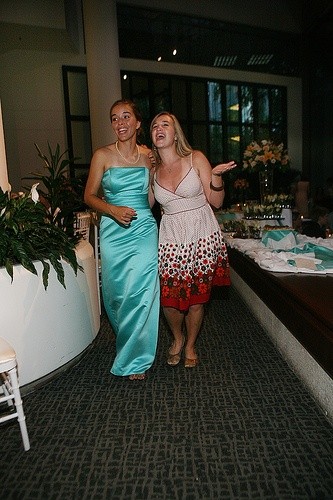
[223,234,333,425]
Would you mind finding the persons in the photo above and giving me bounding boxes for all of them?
[85,99,161,380]
[97,112,237,368]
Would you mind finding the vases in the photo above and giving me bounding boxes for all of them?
[259,166,273,204]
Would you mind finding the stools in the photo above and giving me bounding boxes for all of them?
[0,338,30,451]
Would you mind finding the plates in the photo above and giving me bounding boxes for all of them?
[263,227,296,231]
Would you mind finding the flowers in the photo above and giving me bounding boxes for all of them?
[241,139,289,168]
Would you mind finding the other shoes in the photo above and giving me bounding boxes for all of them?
[129,374,145,381]
[184,358,199,367]
[167,336,185,365]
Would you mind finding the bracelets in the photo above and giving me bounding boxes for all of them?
[210,181,224,191]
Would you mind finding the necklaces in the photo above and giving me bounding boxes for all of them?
[115,140,140,164]
